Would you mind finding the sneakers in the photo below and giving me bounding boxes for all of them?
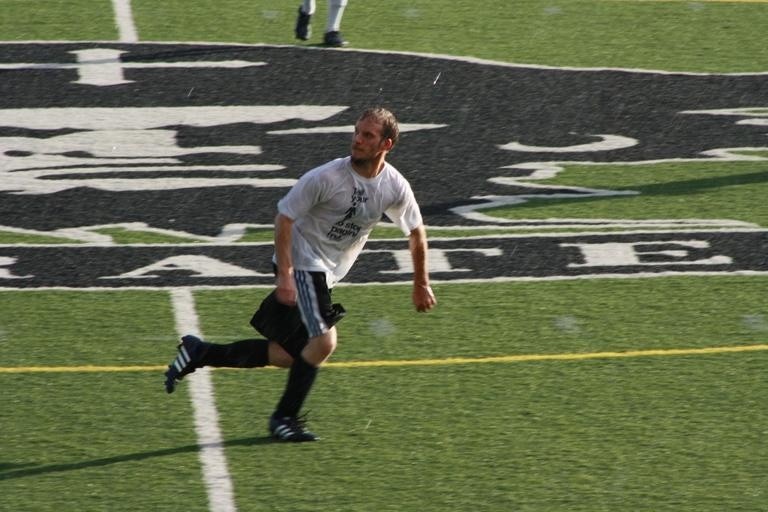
[270,415,318,441]
[164,335,201,394]
[324,31,343,47]
[296,6,312,40]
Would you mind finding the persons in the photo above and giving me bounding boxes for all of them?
[296,0,349,46]
[166,106,436,442]
